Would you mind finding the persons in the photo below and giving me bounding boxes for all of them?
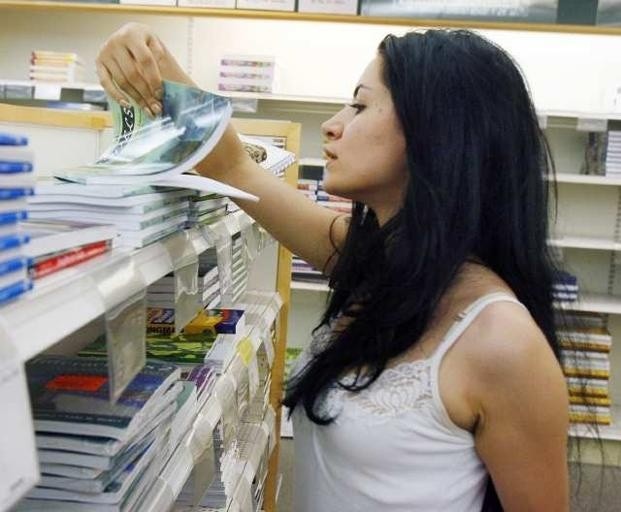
[95,23,619,511]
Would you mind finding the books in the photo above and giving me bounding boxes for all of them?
[290,179,355,279]
[117,1,359,15]
[28,52,88,83]
[284,348,308,382]
[219,54,276,96]
[1,83,292,511]
[550,310,614,426]
[548,265,581,300]
[585,127,621,179]
